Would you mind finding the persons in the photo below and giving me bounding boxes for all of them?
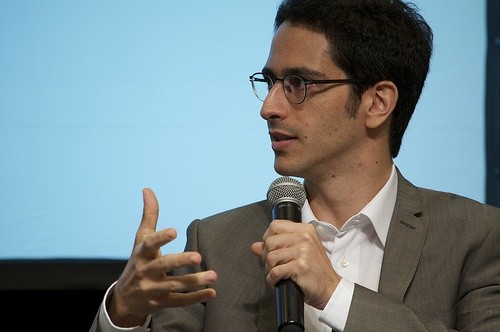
[89,0,500,332]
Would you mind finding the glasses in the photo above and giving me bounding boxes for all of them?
[249,72,367,104]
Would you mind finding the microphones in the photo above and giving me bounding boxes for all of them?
[267,177,308,332]
